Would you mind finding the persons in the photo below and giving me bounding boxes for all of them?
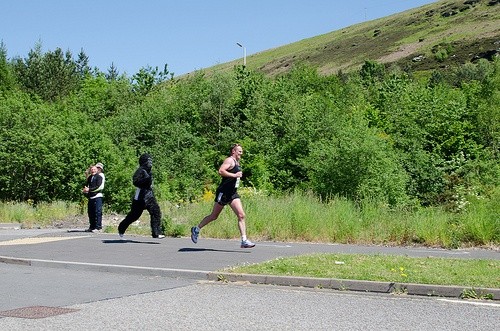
[191,144,255,248]
[118,153,165,239]
[82,163,106,233]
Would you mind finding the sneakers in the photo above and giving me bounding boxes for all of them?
[241,240,255,248]
[191,227,199,243]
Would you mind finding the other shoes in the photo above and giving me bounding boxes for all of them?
[85,229,94,232]
[158,235,165,239]
[92,228,102,233]
[119,234,123,237]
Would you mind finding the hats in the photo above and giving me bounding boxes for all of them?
[230,144,240,153]
[96,163,103,169]
